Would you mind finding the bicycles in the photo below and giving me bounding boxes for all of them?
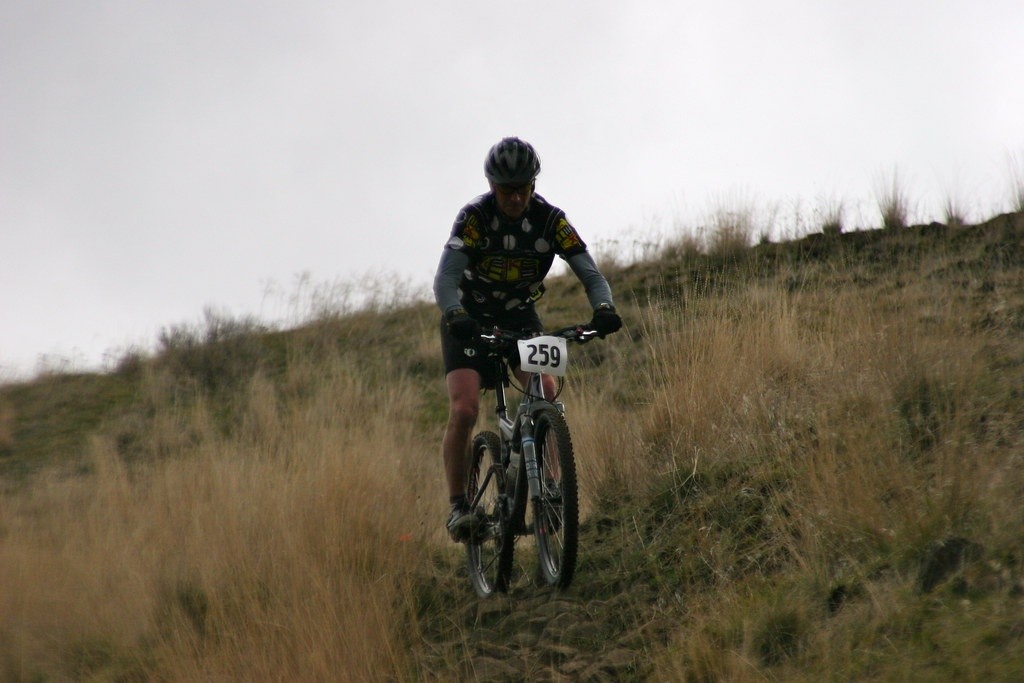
[440,319,597,602]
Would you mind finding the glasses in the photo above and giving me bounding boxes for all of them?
[496,183,532,195]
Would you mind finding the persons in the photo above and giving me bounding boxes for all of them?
[432,136,622,536]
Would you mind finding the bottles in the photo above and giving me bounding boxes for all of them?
[503,452,520,499]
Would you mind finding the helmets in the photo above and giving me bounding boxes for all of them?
[485,137,540,186]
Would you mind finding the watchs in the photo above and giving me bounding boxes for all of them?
[598,303,610,310]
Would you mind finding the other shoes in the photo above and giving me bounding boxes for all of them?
[445,501,479,543]
[545,478,562,534]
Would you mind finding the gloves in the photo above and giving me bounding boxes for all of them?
[584,309,622,340]
[448,314,482,344]
